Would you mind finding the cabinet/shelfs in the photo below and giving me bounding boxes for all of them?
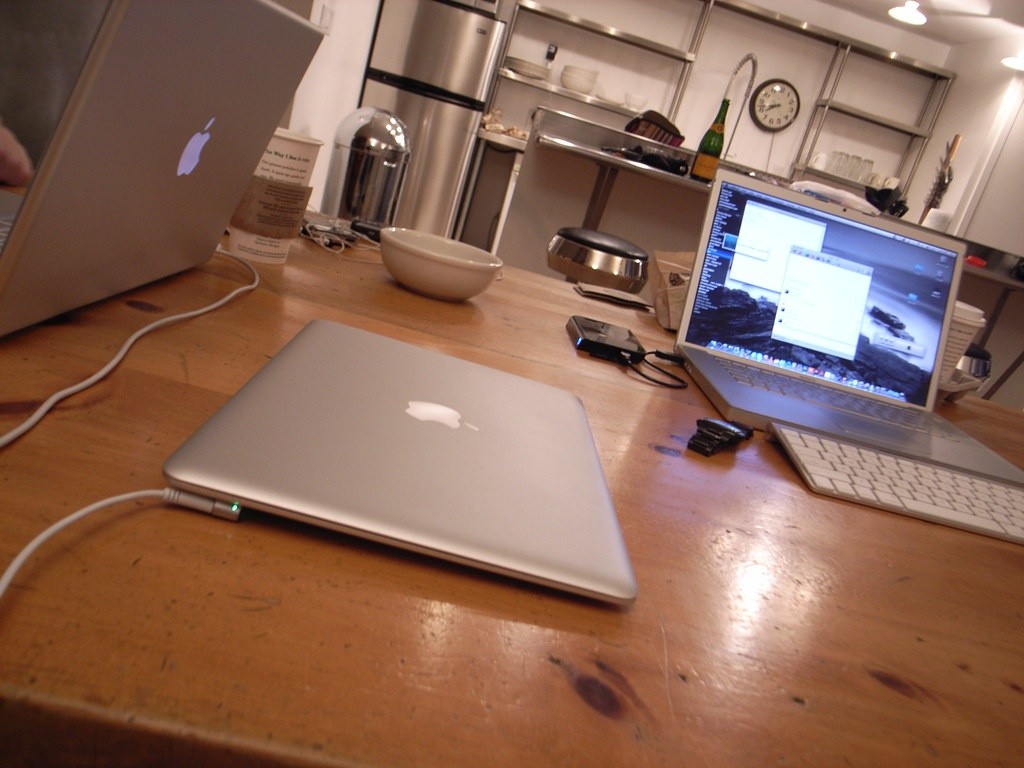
[478,1,959,218]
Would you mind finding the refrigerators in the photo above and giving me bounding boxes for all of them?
[338,0,507,241]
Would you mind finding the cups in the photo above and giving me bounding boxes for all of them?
[825,150,900,189]
[810,151,828,171]
[940,300,986,384]
[922,208,951,232]
[229,127,324,263]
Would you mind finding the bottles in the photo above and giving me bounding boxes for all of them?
[690,98,730,184]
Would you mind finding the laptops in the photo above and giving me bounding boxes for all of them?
[1,2,329,337]
[160,319,638,608]
[673,167,1024,490]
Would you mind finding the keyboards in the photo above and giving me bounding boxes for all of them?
[762,422,1024,546]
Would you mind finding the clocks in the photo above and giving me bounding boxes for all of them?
[749,79,801,133]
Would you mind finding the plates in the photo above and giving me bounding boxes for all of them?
[505,56,550,80]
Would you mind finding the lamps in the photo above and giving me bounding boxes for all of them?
[888,0,927,26]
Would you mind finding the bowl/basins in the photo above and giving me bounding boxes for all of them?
[381,227,503,301]
[561,65,598,93]
[626,93,648,112]
